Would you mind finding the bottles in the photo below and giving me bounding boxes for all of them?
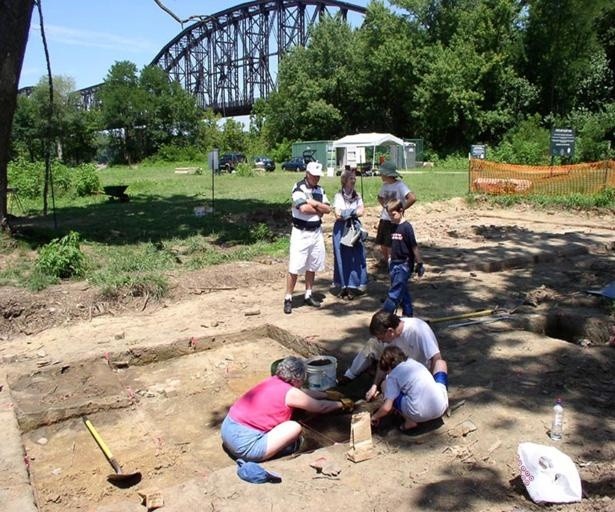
[551,399,564,440]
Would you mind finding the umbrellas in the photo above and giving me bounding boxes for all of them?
[585,282,615,301]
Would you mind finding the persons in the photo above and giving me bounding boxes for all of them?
[373,161,416,269]
[283,162,331,313]
[336,308,442,403]
[221,355,353,463]
[381,199,425,316]
[370,345,449,436]
[332,171,369,298]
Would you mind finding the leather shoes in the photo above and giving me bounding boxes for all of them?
[304,296,320,307]
[284,299,292,313]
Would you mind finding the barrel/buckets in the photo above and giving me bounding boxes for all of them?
[302,355,337,392]
[327,167,334,177]
[194,206,206,217]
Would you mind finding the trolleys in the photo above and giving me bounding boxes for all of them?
[90,184,131,204]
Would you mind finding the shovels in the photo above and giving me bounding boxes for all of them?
[83,415,137,479]
[423,300,525,323]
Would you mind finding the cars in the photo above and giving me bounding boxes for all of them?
[250,155,276,172]
[281,149,318,173]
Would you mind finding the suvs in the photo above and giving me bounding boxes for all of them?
[216,155,248,174]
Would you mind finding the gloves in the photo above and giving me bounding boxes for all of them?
[415,262,424,276]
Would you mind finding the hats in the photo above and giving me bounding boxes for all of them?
[380,163,402,177]
[237,458,282,483]
[306,161,324,176]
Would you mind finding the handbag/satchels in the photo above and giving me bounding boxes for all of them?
[347,412,373,463]
[340,218,361,247]
